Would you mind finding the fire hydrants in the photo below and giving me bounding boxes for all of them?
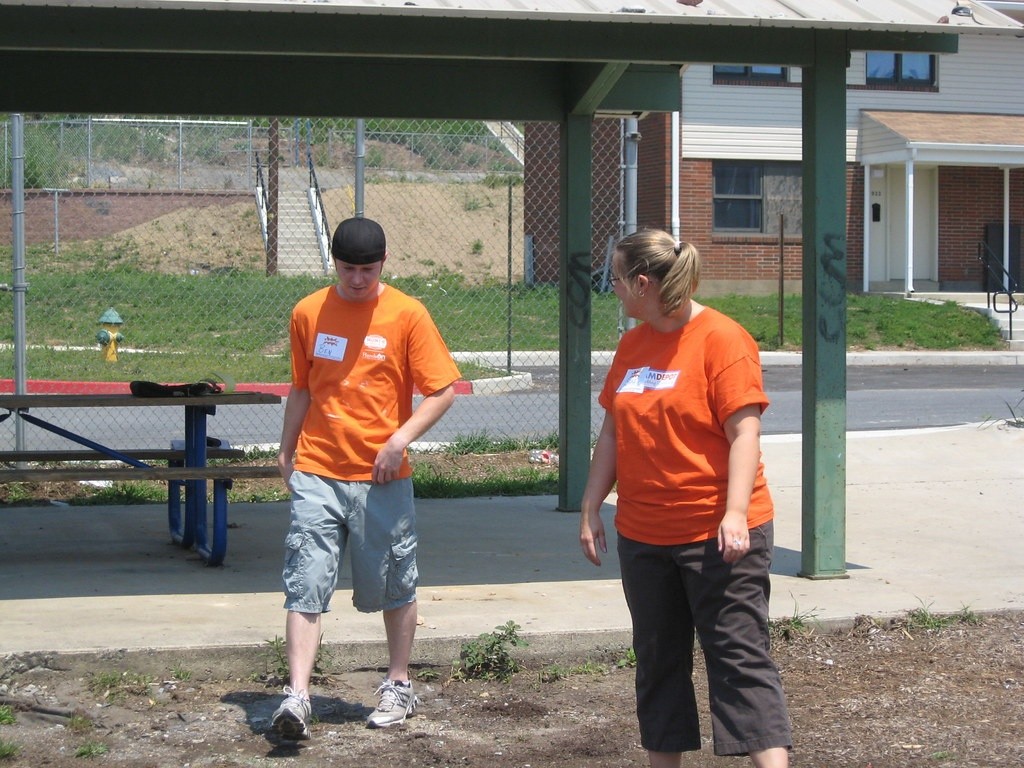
[95,307,125,363]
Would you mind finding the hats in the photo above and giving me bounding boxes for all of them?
[332,217,386,264]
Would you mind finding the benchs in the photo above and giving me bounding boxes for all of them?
[0,447,281,566]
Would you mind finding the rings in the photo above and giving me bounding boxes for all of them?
[733,539,740,545]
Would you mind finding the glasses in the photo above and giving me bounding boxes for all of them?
[608,273,639,286]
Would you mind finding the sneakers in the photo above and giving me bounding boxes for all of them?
[271,694,313,741]
[366,679,419,727]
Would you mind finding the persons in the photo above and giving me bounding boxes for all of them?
[580,228,793,768]
[269,217,463,740]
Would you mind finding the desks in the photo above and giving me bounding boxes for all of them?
[0,391,282,568]
